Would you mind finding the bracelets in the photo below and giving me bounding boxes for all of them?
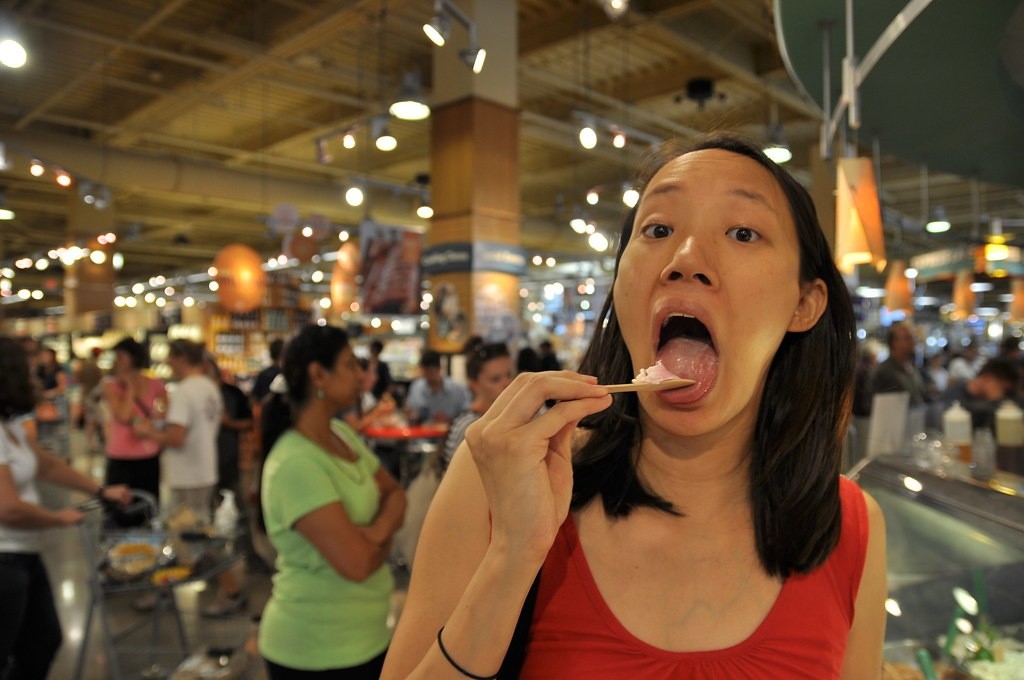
[438,627,489,680]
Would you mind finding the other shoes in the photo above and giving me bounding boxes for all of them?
[132,593,173,610]
[201,592,248,618]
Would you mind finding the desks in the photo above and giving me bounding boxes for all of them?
[361,422,450,483]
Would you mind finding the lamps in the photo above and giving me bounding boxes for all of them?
[761,119,793,164]
[422,0,487,75]
[388,71,432,122]
[925,204,951,233]
[568,107,628,149]
[375,126,398,152]
[569,218,609,253]
[343,128,356,149]
[0,15,31,67]
[834,111,888,275]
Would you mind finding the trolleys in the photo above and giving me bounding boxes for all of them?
[32,387,73,464]
[72,488,251,680]
[85,404,103,474]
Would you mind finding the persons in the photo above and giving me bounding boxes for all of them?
[853,322,1024,434]
[255,323,408,680]
[252,336,564,482]
[377,131,888,680]
[0,334,274,680]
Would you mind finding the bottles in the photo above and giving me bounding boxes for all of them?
[993,399,1024,476]
[941,401,973,468]
